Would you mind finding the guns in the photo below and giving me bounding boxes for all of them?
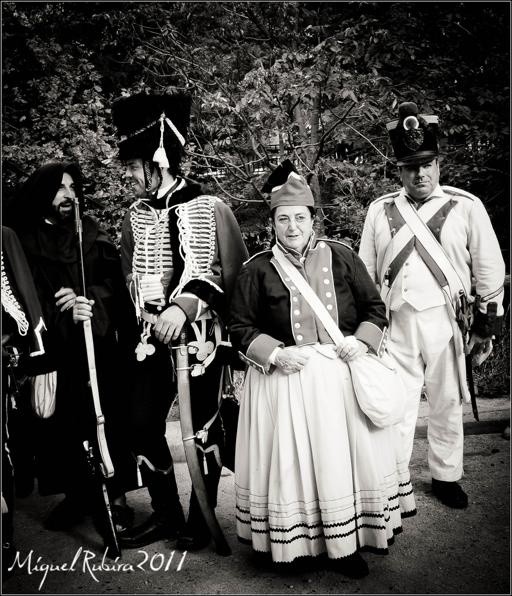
[71,197,125,561]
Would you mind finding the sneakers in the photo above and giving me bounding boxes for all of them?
[429,476,469,509]
[337,554,368,580]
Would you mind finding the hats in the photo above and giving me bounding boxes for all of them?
[112,91,196,165]
[258,159,315,209]
[386,100,439,167]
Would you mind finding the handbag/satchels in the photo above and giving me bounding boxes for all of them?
[347,345,407,428]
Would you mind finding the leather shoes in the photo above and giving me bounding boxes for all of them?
[118,508,186,549]
[177,510,210,552]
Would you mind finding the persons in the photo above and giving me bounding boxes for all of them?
[352,102,506,510]
[230,172,416,579]
[111,85,247,552]
[2,163,134,536]
